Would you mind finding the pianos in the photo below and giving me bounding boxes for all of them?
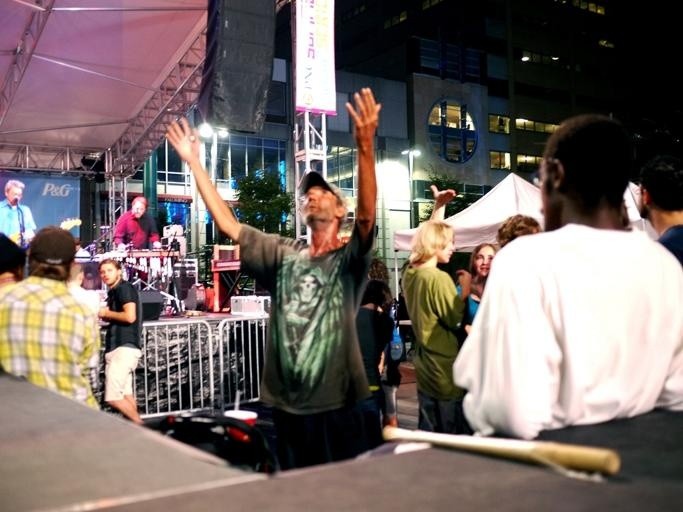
[110,249,180,258]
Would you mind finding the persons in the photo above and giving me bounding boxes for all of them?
[0,179,35,255]
[0,227,102,413]
[0,235,26,289]
[98,260,145,424]
[451,113,683,439]
[111,196,162,291]
[396,185,542,435]
[355,257,405,428]
[72,236,92,263]
[67,262,100,316]
[629,130,683,266]
[161,84,384,469]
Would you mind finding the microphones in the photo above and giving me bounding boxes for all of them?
[134,209,141,215]
[13,196,19,206]
[114,202,123,213]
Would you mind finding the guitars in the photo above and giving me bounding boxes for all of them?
[9,217,82,256]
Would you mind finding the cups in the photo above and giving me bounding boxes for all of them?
[222,409,256,440]
[230,296,264,317]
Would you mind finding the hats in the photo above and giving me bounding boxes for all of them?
[297,171,346,204]
[30,228,76,266]
[0,232,25,271]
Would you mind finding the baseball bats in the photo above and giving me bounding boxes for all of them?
[382,424,621,475]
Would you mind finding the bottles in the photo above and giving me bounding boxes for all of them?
[390,335,403,360]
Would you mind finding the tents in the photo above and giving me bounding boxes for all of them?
[624,181,660,241]
[395,172,545,301]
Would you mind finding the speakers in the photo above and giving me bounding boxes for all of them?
[193,0,277,134]
[136,289,165,319]
